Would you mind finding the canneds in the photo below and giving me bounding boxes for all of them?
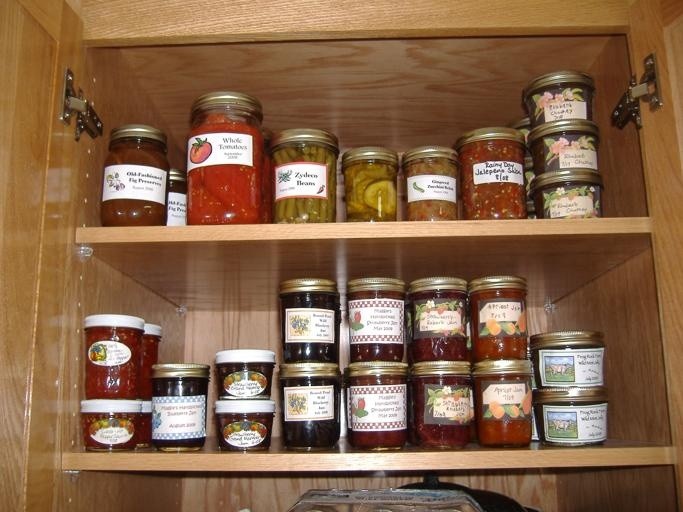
[213,348,276,400]
[276,361,341,446]
[341,146,399,222]
[405,274,469,363]
[531,388,612,445]
[346,278,409,361]
[348,361,411,447]
[185,90,265,225]
[521,69,596,129]
[143,323,165,398]
[530,329,607,389]
[402,145,464,222]
[469,360,533,447]
[409,363,473,450]
[470,275,528,359]
[101,122,170,227]
[84,314,144,399]
[149,363,211,452]
[276,276,340,363]
[78,400,142,452]
[268,126,340,224]
[454,126,527,220]
[529,168,602,218]
[529,118,600,177]
[215,401,276,450]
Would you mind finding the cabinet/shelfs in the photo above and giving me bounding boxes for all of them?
[0,1,683,512]
